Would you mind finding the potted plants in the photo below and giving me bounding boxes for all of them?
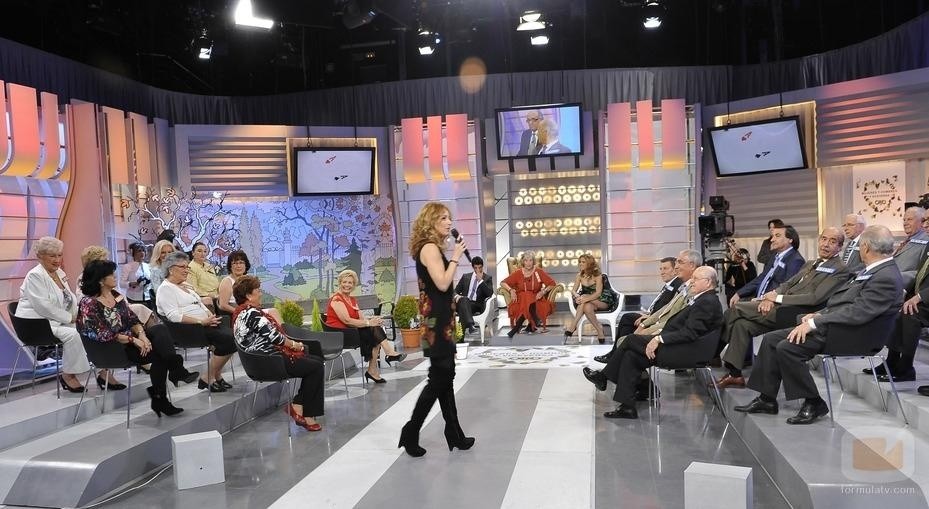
[391,295,420,348]
[453,321,469,360]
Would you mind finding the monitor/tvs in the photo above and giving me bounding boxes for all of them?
[706,115,810,178]
[293,145,375,196]
[494,102,586,161]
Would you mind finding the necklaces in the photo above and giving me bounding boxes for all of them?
[46,269,58,282]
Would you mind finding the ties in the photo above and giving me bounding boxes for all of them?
[469,275,477,300]
[844,240,854,263]
[804,258,822,278]
[758,256,777,296]
[529,132,536,154]
[540,145,547,154]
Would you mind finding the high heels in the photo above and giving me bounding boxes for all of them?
[197,371,233,391]
[385,352,408,366]
[59,365,150,393]
[365,370,386,383]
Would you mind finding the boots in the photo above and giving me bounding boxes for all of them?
[437,377,475,451]
[399,378,437,456]
[147,384,183,416]
[168,353,199,386]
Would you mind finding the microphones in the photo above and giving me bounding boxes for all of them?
[450,228,471,259]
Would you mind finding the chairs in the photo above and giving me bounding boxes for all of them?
[564,273,625,343]
[496,256,564,333]
[647,351,910,427]
[455,293,496,344]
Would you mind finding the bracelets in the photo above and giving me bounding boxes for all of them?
[127,335,133,345]
[290,340,297,352]
[136,330,145,336]
[366,320,370,327]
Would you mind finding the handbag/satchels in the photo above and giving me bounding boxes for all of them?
[284,348,305,357]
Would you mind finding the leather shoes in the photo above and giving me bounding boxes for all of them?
[583,354,660,419]
[705,357,829,423]
[469,323,605,344]
[284,403,322,431]
[862,357,929,397]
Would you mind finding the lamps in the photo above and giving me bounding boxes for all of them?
[175,0,215,62]
[365,48,377,62]
[409,0,440,58]
[513,0,553,49]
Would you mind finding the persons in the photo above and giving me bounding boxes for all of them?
[397,203,475,458]
[514,110,573,156]
[453,256,493,343]
[584,207,928,424]
[564,254,617,343]
[77,259,200,418]
[230,276,325,430]
[325,269,407,384]
[500,250,556,337]
[154,251,234,394]
[15,235,127,393]
[73,237,251,329]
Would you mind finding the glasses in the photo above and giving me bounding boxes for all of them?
[527,118,539,123]
[173,264,192,271]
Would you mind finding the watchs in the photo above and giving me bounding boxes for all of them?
[449,259,460,266]
